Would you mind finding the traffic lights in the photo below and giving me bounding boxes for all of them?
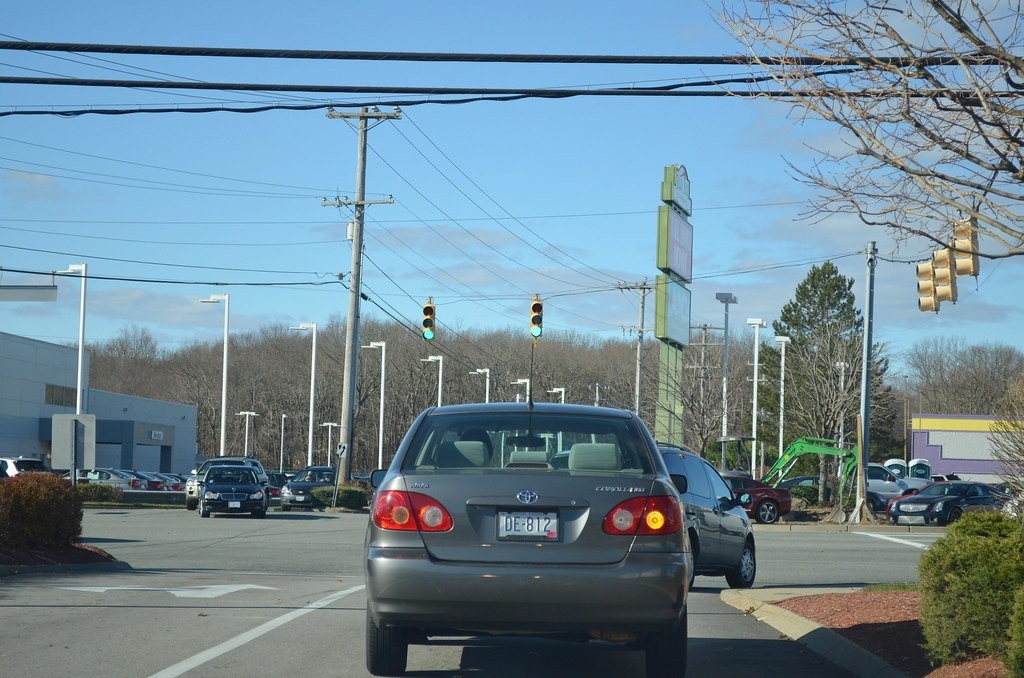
[916,218,980,313]
[530,301,543,337]
[423,304,436,339]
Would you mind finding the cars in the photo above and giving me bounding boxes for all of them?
[363,399,792,678]
[866,463,1013,529]
[185,456,337,518]
[0,455,193,492]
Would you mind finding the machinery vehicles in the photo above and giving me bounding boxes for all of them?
[762,436,859,524]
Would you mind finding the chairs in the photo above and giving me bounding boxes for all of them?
[239,473,250,482]
[437,441,489,467]
[214,473,224,482]
[311,474,316,482]
[567,442,622,470]
[510,451,548,463]
[325,474,333,482]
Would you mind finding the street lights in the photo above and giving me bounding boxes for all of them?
[835,361,850,475]
[200,293,230,456]
[57,263,87,414]
[360,340,386,468]
[547,386,565,403]
[420,355,443,408]
[747,317,768,480]
[289,322,318,475]
[775,336,792,476]
[236,410,259,458]
[715,292,739,469]
[319,422,342,468]
[511,377,530,403]
[468,368,490,403]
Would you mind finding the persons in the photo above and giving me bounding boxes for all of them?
[306,474,313,481]
